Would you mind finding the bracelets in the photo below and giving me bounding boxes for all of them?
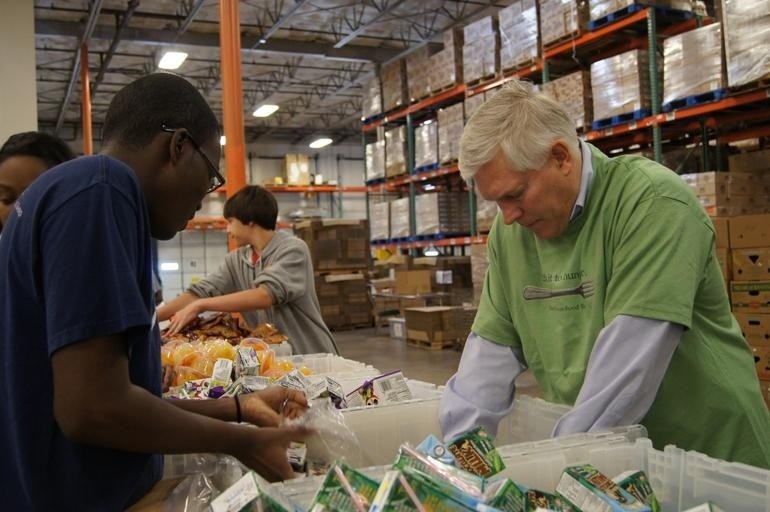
[233,389,245,426]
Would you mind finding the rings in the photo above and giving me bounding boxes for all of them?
[280,396,289,411]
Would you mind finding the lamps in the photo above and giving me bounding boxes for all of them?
[251,104,280,118]
[158,49,188,71]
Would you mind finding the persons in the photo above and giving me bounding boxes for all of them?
[426,75,770,471]
[1,71,323,511]
[0,131,81,224]
[136,180,342,354]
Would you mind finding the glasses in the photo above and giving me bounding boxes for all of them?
[156,121,226,195]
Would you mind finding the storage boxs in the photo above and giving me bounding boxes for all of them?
[282,151,311,187]
[662,144,769,405]
[160,330,770,510]
[373,241,490,356]
[294,216,371,331]
